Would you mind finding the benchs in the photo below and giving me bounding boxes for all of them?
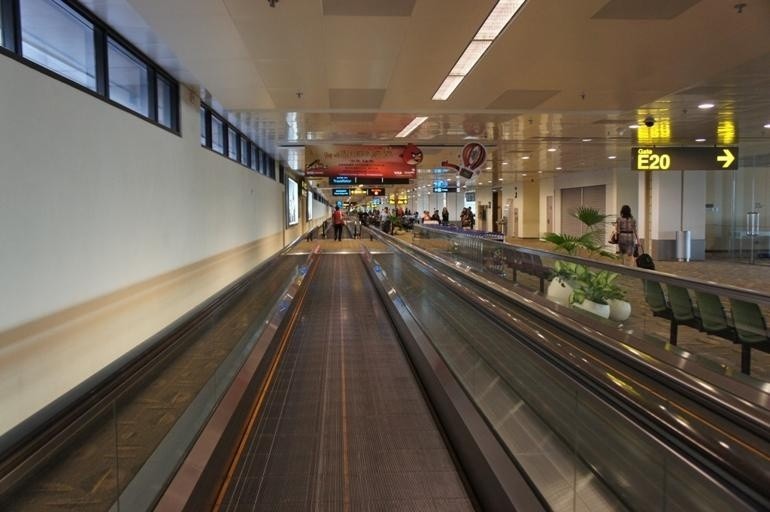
[639,270,770,375]
[501,247,552,293]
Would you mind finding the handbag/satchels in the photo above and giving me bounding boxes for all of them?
[608,223,619,244]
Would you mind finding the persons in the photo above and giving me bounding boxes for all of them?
[460,206,475,230]
[441,207,449,226]
[332,207,441,242]
[612,205,641,267]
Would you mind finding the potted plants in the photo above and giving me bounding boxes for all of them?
[541,206,631,323]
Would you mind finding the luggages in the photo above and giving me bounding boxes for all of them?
[633,243,656,270]
